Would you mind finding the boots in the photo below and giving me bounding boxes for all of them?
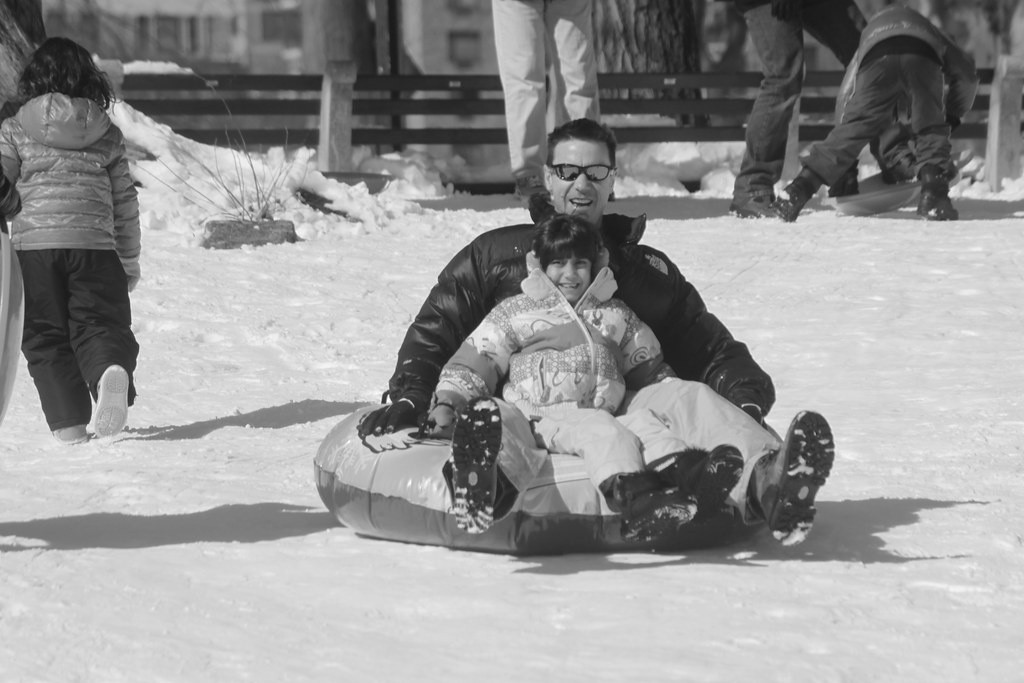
[770,175,815,223]
[916,164,959,222]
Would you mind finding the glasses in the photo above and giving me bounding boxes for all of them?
[546,163,614,182]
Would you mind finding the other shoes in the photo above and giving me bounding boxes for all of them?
[882,156,919,187]
[52,423,89,446]
[92,364,130,438]
[660,444,744,521]
[748,410,835,547]
[515,177,551,210]
[730,194,777,218]
[828,166,858,198]
[614,469,699,543]
[450,397,503,535]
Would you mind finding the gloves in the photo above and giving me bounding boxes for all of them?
[739,397,770,432]
[118,255,140,293]
[356,393,427,454]
[945,113,962,131]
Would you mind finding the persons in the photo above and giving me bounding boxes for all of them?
[359,117,836,547]
[0,36,142,447]
[491,0,616,211]
[729,0,980,221]
